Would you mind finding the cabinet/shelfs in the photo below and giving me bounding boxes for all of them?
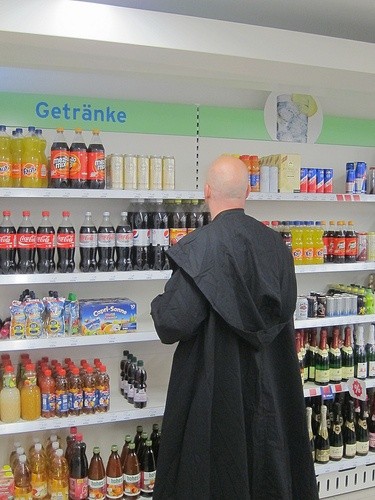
[1,189,375,500]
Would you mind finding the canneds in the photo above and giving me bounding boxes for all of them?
[294,292,358,320]
[107,153,176,191]
[344,161,367,194]
[259,166,279,193]
[357,231,375,261]
[300,167,333,193]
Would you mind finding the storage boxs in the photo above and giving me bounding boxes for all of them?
[79,297,137,335]
[260,153,300,193]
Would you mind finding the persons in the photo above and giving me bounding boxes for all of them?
[151,156,320,500]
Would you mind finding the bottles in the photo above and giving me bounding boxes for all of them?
[0,198,212,276]
[9,423,162,500]
[344,162,375,195]
[0,124,107,191]
[294,324,375,387]
[260,220,358,265]
[221,152,261,192]
[118,350,152,409]
[304,388,375,465]
[326,283,375,315]
[0,353,112,423]
